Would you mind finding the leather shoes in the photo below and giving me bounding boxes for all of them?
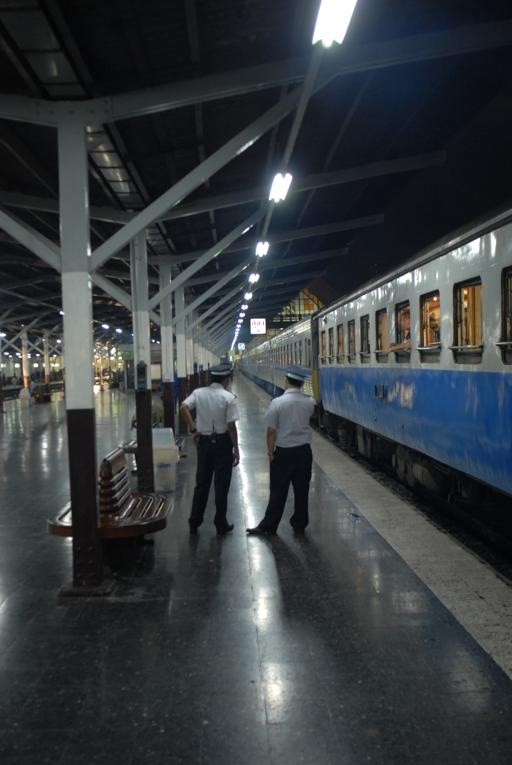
[217,523,233,534]
[247,527,275,535]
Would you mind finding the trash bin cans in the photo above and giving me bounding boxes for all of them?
[18,388,31,410]
[153,428,177,493]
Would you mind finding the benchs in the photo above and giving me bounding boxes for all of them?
[46,447,173,577]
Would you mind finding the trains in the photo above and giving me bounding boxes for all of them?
[237,205,512,569]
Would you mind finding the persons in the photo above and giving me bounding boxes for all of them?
[181,364,239,535]
[30,378,36,397]
[246,369,315,536]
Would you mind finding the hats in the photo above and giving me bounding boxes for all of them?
[286,365,308,381]
[209,362,232,377]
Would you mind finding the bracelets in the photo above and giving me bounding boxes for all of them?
[189,428,197,433]
[268,452,274,456]
